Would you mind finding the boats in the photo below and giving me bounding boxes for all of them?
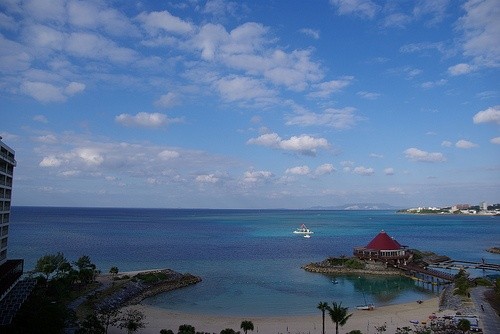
[303,234,310,238]
[356,304,375,310]
